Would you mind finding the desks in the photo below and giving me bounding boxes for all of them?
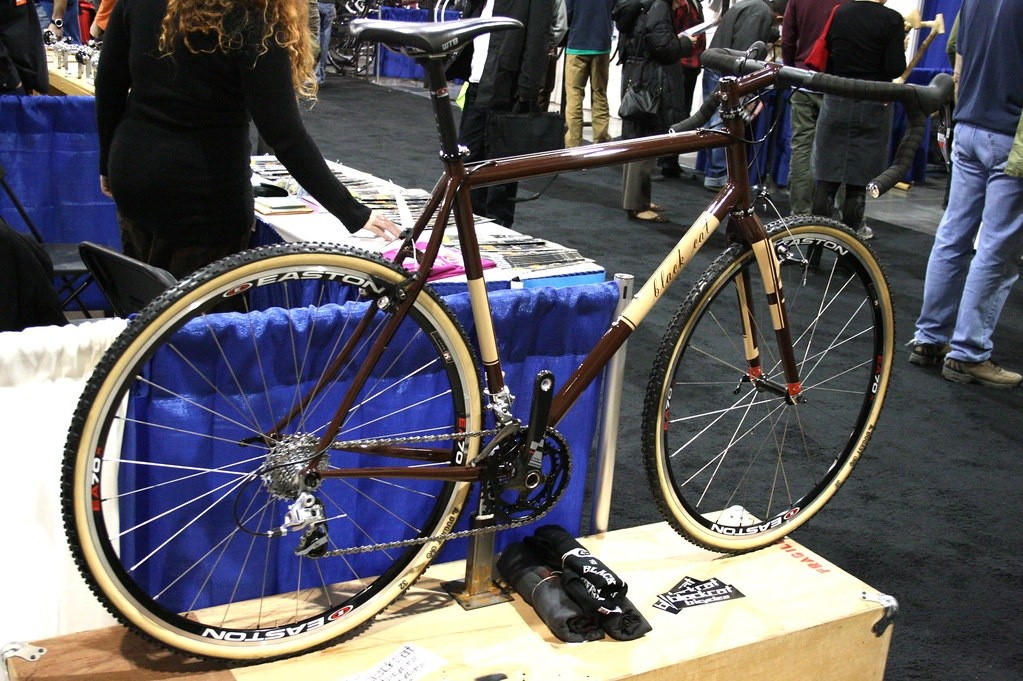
[246,154,605,312]
[44,42,101,96]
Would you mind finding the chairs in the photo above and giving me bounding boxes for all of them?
[0,162,207,332]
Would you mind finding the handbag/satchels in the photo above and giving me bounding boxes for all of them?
[484,95,565,159]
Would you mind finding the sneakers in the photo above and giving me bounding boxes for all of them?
[942,357,1022,388]
[908,344,952,366]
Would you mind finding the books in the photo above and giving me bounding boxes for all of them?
[253,197,314,216]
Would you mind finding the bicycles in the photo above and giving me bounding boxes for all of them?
[61,14,959,665]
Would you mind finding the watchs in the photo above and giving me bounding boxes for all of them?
[51,19,64,29]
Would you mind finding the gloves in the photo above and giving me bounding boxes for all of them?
[513,88,537,111]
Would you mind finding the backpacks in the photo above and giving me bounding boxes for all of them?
[802,5,841,73]
[618,83,662,122]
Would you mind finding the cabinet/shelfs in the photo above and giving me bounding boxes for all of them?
[0,503,899,681]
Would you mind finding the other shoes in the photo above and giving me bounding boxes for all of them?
[863,225,873,240]
[655,167,696,180]
[627,202,666,222]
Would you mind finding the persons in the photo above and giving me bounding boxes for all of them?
[621,0,699,223]
[94,0,402,282]
[310,0,336,86]
[810,1,906,240]
[905,0,1023,390]
[695,0,787,196]
[446,0,569,229]
[781,0,854,218]
[656,1,706,181]
[33,0,117,50]
[565,0,614,149]
